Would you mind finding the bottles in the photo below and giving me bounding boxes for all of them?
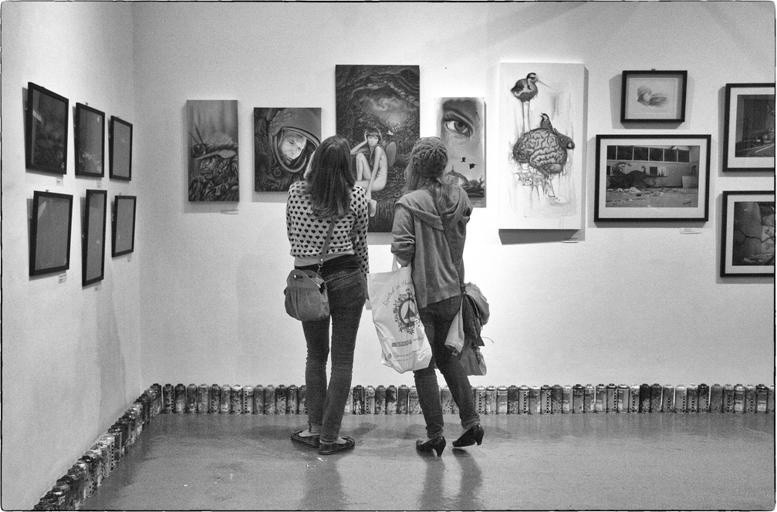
[161,384,773,415]
[34,382,162,510]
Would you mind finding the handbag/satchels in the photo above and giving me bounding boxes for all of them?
[282,268,331,323]
[366,256,431,373]
[462,296,487,377]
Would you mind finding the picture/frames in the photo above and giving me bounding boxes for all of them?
[26,82,69,174]
[82,188,108,287]
[719,189,775,277]
[29,190,73,276]
[112,194,136,257]
[620,69,688,123]
[723,82,775,173]
[593,133,712,223]
[109,114,133,181]
[74,102,106,177]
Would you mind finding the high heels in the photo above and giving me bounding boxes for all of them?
[414,435,448,458]
[450,423,486,451]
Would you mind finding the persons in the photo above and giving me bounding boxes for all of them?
[284,135,369,455]
[437,97,484,183]
[279,132,307,166]
[349,127,388,217]
[391,136,484,459]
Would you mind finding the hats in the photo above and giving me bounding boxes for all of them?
[409,136,449,180]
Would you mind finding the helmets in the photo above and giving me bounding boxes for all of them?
[269,108,320,173]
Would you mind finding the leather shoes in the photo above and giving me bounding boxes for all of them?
[289,429,322,448]
[319,435,356,457]
